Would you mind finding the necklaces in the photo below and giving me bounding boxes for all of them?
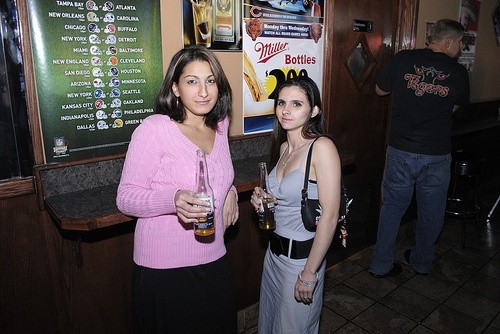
[282,148,295,169]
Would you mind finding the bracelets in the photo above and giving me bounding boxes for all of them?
[297,269,319,286]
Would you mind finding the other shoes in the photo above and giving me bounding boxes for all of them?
[405,248,423,276]
[370,261,401,278]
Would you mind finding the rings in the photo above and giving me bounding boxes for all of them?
[304,297,308,299]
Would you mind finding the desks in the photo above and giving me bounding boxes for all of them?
[454,100,500,150]
[34,132,356,267]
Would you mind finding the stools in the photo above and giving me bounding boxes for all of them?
[435,155,487,247]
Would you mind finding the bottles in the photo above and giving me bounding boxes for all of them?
[256,162,275,230]
[192,149,215,243]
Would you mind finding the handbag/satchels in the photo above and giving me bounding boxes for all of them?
[301,135,346,232]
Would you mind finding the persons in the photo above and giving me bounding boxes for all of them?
[115,45,240,334]
[250,76,343,334]
[368,19,472,278]
